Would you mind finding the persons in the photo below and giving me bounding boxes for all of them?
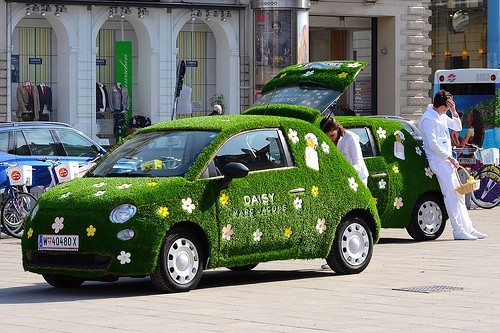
[419,89,488,240]
[320,115,369,272]
[452,109,485,171]
[14,80,128,131]
[263,20,291,64]
[210,104,224,118]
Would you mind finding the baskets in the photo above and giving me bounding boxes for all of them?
[456,165,480,194]
[6,165,32,187]
[54,162,79,184]
[480,148,500,166]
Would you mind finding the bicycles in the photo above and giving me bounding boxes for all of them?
[0,161,40,240]
[33,157,80,191]
[464,142,500,182]
[447,150,500,210]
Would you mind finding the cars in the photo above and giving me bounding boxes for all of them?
[19,59,382,294]
[0,119,109,206]
[320,115,450,243]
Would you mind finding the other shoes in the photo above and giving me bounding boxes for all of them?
[320,264,330,269]
[454,231,479,240]
[471,230,487,239]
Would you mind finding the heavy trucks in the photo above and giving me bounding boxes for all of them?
[432,67,500,182]
